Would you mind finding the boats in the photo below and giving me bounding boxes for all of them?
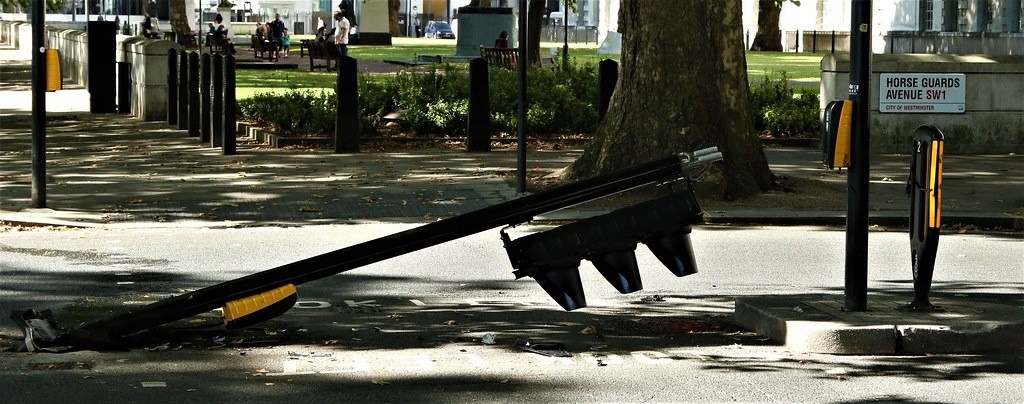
[221,283,300,331]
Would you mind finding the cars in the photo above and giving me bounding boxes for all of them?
[424,20,456,40]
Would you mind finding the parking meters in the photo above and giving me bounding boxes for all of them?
[45,49,64,92]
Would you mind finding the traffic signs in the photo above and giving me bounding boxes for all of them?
[823,100,854,167]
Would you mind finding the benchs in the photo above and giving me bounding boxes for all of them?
[251,36,282,61]
[307,40,348,73]
[481,45,519,69]
[299,39,315,58]
[207,35,230,55]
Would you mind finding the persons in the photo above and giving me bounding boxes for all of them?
[96,9,292,63]
[350,24,360,45]
[333,10,350,70]
[413,17,421,37]
[494,30,510,49]
[324,27,337,59]
[314,27,326,43]
[317,17,325,36]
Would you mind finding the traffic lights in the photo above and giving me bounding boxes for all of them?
[507,193,699,312]
